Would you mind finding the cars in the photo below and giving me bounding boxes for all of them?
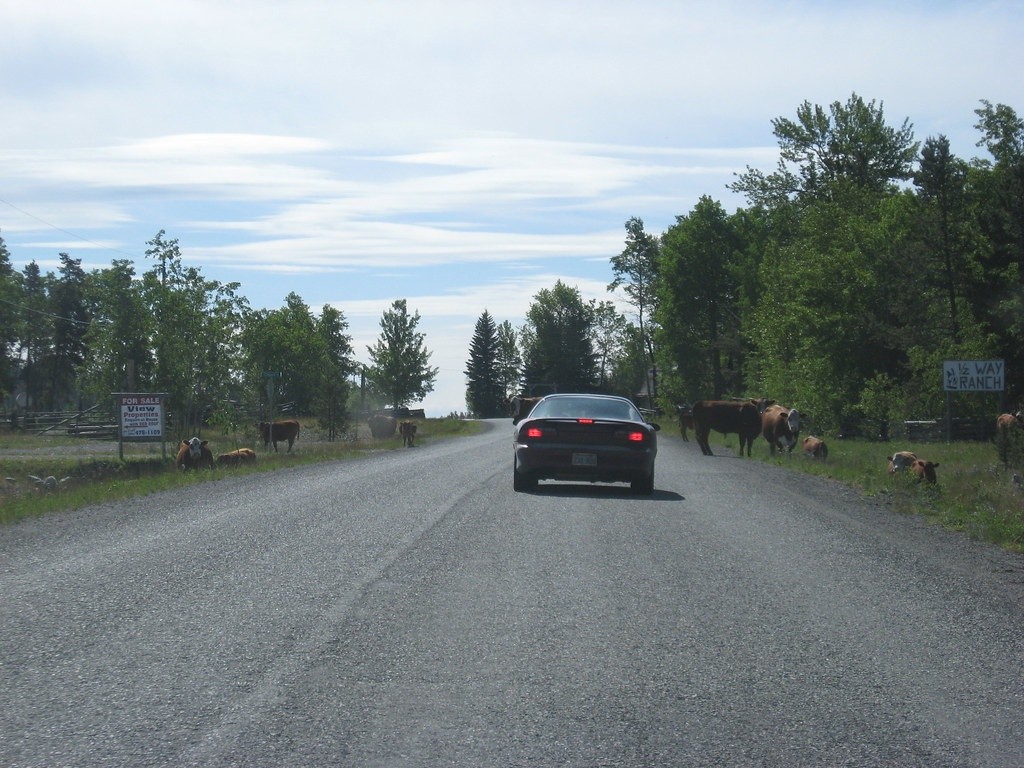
[511,395,663,493]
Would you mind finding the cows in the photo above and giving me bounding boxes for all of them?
[503,395,543,426]
[216,447,255,468]
[398,421,417,446]
[366,414,399,439]
[176,437,214,473]
[257,420,300,453]
[997,412,1024,433]
[679,397,827,462]
[887,451,940,484]
